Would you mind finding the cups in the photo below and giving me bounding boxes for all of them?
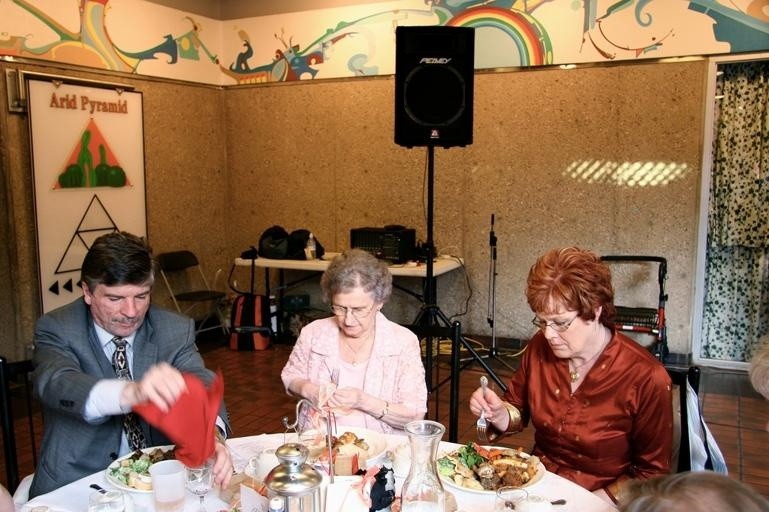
[495,485,529,511]
[186,462,215,511]
[148,459,186,504]
[88,489,125,512]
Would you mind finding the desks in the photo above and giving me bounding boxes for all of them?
[234,250,467,301]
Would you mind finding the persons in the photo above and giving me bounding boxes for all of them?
[471,249,674,512]
[27,232,234,502]
[280,248,427,436]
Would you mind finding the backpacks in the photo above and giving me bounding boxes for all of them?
[228,293,273,351]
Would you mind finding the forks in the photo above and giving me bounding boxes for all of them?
[476,375,489,441]
[332,368,341,388]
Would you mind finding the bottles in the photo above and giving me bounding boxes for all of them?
[307,232,315,260]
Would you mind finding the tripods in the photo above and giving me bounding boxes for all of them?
[408,147,510,393]
[456,213,521,375]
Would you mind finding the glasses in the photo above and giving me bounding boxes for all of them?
[328,298,376,318]
[530,314,578,332]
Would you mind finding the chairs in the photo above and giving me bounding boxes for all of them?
[599,252,667,364]
[662,361,727,480]
[385,321,463,443]
[154,251,229,339]
[0,356,40,499]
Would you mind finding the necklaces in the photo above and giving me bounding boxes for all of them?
[338,332,372,357]
[568,326,607,383]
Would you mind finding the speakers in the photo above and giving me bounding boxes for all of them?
[394,25,475,150]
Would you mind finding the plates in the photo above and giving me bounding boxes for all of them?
[437,444,547,495]
[104,444,177,495]
[297,426,390,462]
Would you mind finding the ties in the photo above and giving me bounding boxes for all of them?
[111,336,146,452]
